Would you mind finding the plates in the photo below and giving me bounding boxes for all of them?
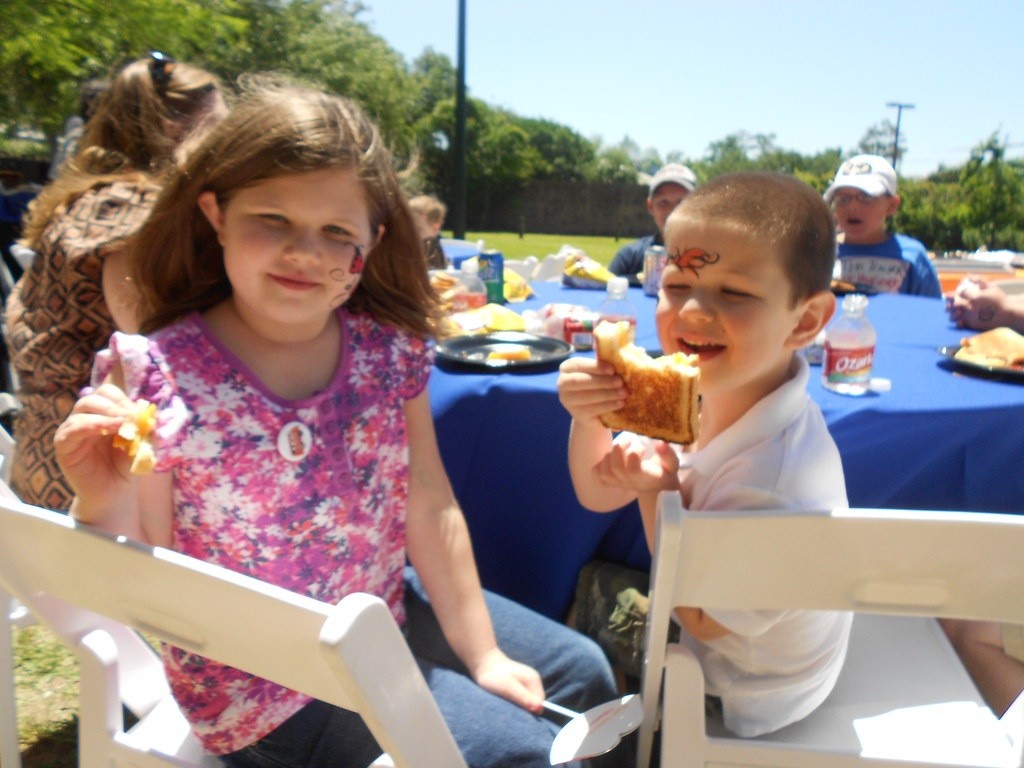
[432,329,575,375]
[935,344,1024,380]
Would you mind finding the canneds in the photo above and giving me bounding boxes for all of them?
[643,245,669,298]
[478,250,505,302]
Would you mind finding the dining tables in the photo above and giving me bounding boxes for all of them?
[428,280,1024,622]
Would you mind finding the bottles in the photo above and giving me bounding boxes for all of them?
[451,262,487,314]
[818,292,878,396]
[597,276,637,321]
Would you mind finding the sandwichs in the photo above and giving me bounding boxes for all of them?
[116,398,164,474]
[594,319,702,446]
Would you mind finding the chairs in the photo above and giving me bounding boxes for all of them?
[1,458,470,768]
[634,490,1023,768]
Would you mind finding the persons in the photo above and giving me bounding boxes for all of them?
[607,164,697,287]
[53,73,635,768]
[944,275,1024,332]
[0,50,229,517]
[556,170,854,738]
[407,196,445,270]
[823,154,941,298]
[940,618,1024,717]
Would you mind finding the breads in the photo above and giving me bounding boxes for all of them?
[960,325,1024,365]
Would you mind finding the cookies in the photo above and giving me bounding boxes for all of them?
[430,269,458,292]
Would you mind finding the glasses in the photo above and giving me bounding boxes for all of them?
[834,192,876,206]
[147,50,168,85]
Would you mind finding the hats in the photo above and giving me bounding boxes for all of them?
[649,164,695,198]
[823,154,896,204]
